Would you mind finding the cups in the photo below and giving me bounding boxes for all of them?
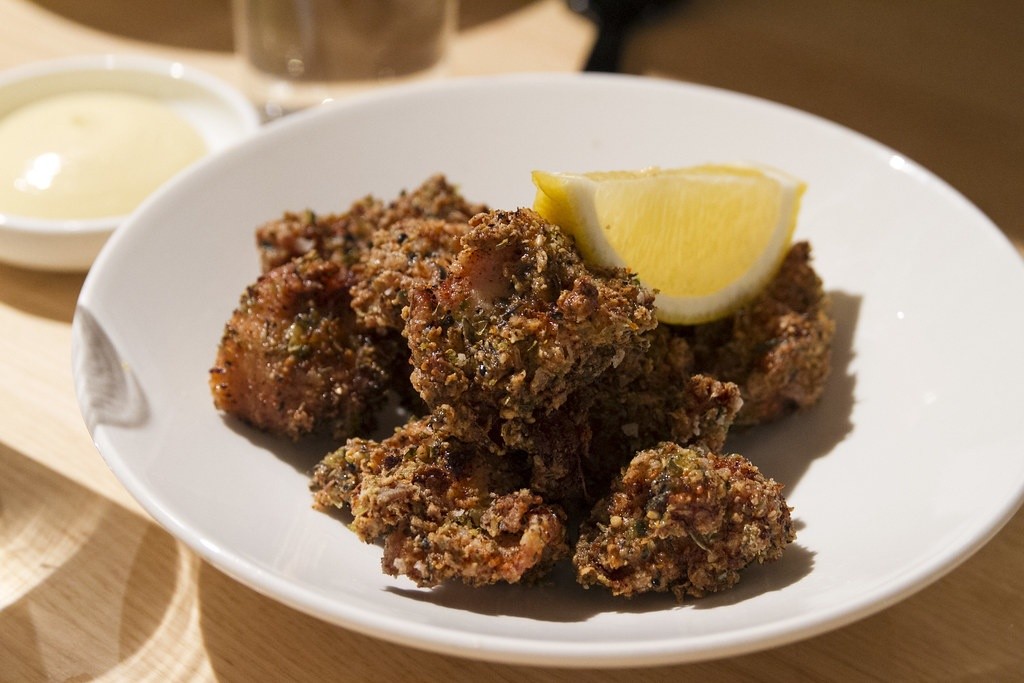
[229,0,457,109]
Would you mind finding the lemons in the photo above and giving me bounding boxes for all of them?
[531,162,807,325]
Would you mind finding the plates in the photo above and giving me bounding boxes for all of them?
[70,72,1023,668]
[1,54,260,274]
